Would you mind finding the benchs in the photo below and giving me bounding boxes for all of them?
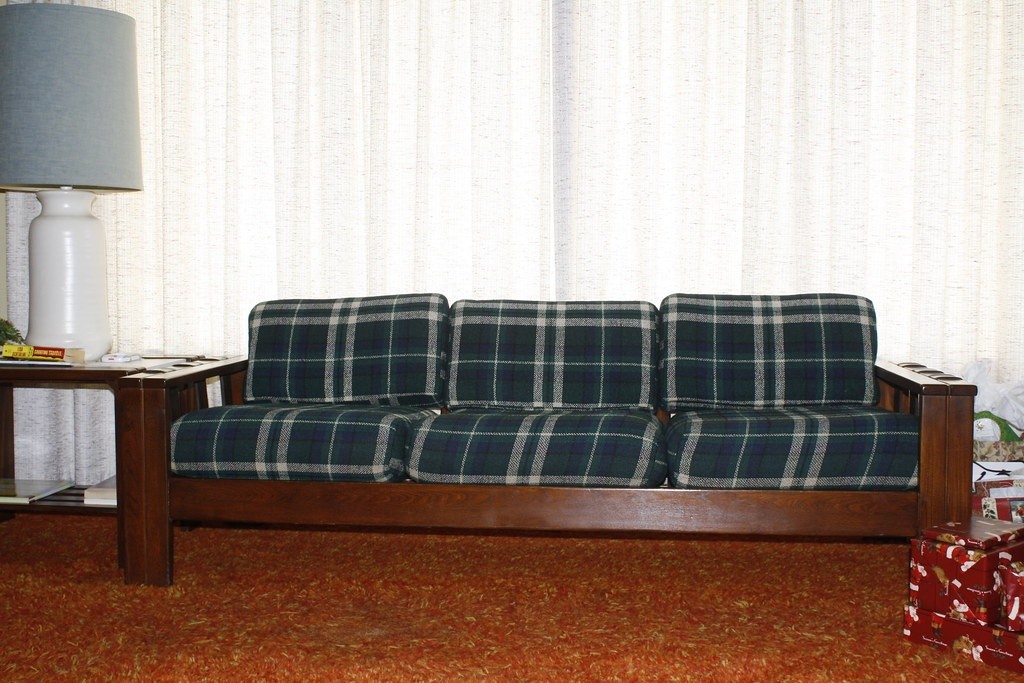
[118,291,980,594]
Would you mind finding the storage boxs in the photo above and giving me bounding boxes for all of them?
[902,462,1024,672]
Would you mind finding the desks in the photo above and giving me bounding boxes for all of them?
[1,355,247,590]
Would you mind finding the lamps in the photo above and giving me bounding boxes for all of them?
[0,2,145,355]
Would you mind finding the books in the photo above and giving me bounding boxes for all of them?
[0,478,75,504]
[84,474,117,505]
[2,345,84,363]
